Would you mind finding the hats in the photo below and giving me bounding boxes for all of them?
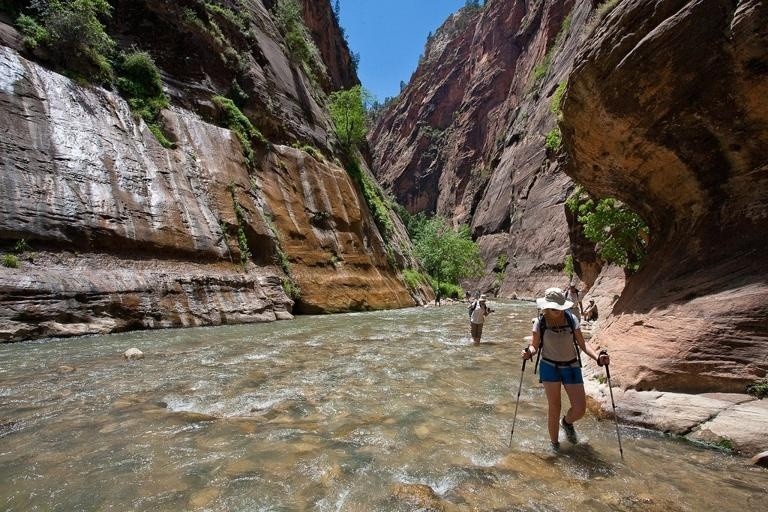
[589,299,595,302]
[479,295,487,301]
[536,288,574,311]
[571,283,575,287]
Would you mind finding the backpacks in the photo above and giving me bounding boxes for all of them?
[469,301,486,316]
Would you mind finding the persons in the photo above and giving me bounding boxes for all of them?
[583,299,598,323]
[467,294,490,344]
[564,282,584,322]
[521,287,610,457]
[434,288,441,307]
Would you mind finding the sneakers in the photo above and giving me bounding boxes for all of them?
[559,416,577,444]
[551,442,560,454]
